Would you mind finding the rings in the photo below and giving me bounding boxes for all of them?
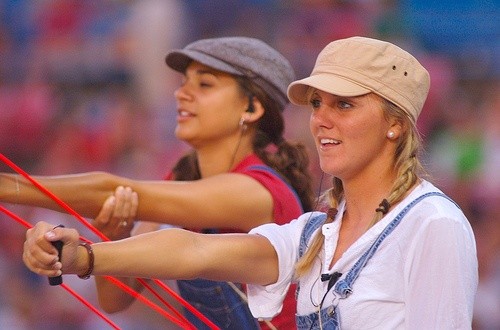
[118,221,127,226]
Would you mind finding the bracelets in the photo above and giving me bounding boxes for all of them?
[77,243,94,280]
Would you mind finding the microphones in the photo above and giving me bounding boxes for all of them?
[321,272,342,291]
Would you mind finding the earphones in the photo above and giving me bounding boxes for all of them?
[246,106,255,113]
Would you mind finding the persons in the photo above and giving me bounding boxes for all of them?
[23,36,479,330]
[0,37,315,329]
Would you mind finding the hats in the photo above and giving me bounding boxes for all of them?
[164,35,297,112]
[287,36,431,127]
